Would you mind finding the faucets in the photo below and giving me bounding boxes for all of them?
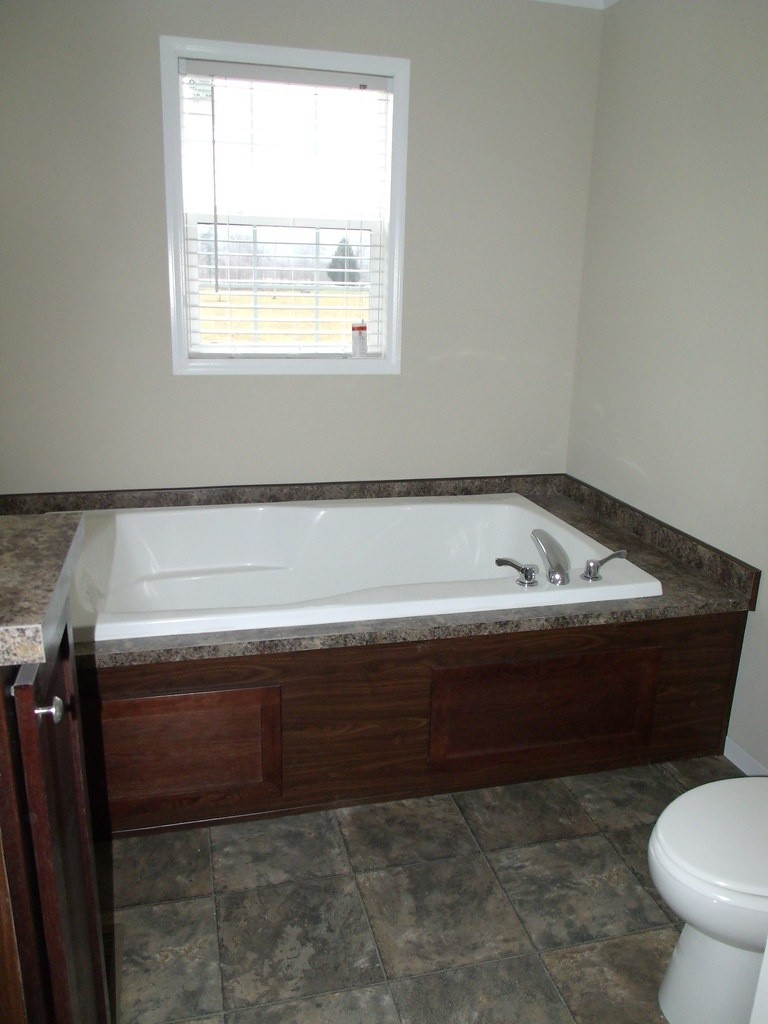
[528,528,572,585]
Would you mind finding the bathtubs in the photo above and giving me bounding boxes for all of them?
[41,486,666,643]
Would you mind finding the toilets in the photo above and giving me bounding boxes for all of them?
[643,773,767,1024]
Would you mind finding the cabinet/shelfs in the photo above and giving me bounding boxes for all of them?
[0,598,115,1024]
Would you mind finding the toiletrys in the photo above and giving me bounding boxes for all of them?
[351,322,368,359]
